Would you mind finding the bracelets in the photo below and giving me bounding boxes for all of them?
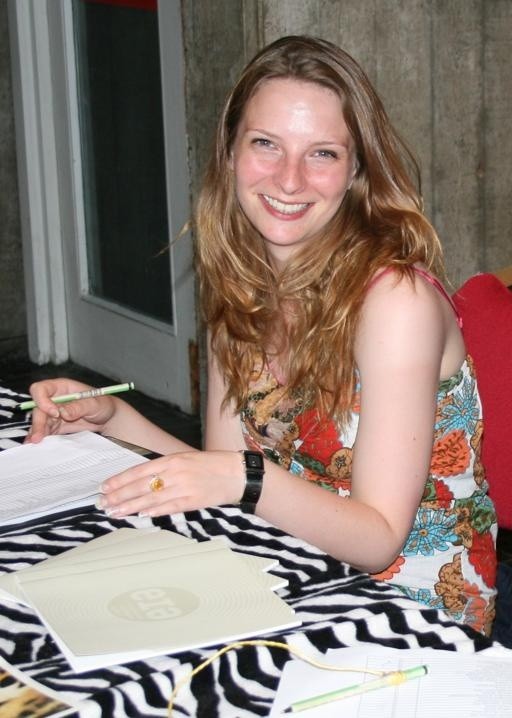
[238,449,265,514]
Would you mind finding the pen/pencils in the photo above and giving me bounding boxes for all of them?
[15,381,136,412]
[280,667,428,714]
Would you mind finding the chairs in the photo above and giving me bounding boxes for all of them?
[452,265,512,530]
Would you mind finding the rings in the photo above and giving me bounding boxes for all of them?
[151,472,164,492]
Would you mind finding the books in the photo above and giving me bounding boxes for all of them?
[0,524,303,674]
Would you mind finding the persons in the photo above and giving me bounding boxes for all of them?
[23,35,501,639]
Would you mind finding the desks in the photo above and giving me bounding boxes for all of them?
[0,390,512,717]
